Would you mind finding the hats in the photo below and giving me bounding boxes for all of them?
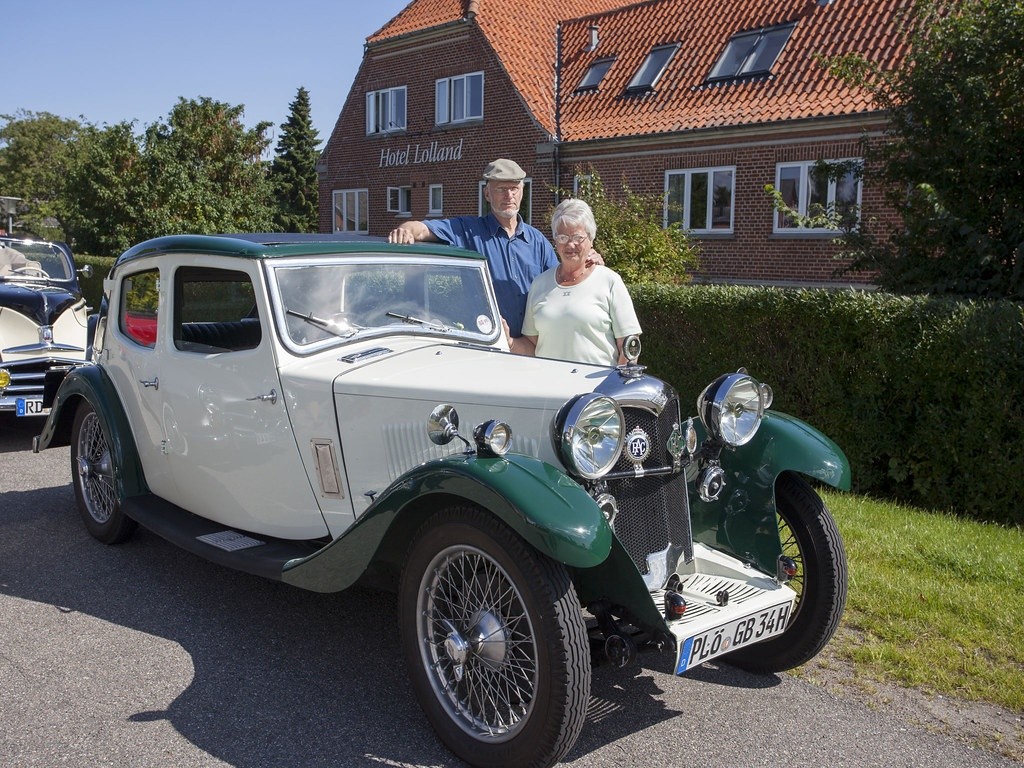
[483,159,526,181]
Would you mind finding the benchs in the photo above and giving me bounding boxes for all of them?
[183,320,262,349]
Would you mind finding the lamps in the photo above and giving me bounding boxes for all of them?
[377,116,448,139]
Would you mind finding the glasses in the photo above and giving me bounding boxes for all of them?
[555,233,589,244]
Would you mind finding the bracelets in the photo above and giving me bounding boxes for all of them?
[510,337,514,351]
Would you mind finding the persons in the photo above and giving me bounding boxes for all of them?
[500,198,643,367]
[388,157,604,339]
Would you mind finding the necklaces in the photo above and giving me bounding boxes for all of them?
[558,264,589,282]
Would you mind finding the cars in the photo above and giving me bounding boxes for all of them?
[0,233,100,424]
[30,231,856,767]
